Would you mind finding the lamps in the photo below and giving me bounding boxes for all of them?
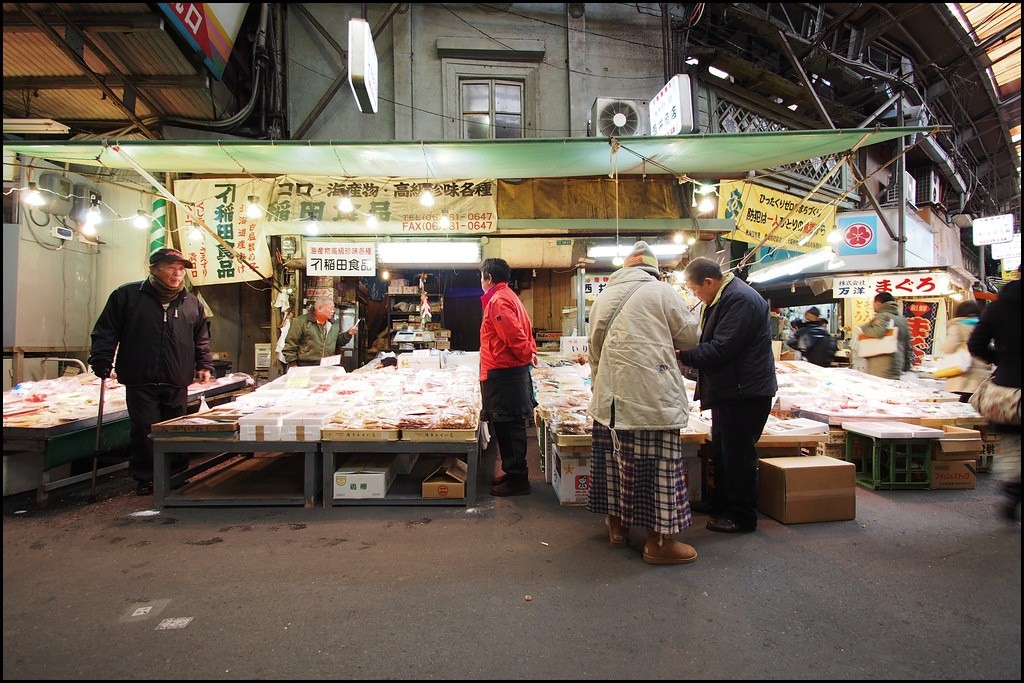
[3,89,71,133]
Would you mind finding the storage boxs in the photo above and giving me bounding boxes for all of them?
[388,278,451,349]
[817,425,1000,492]
[3,451,72,495]
[321,424,478,499]
[306,276,341,302]
[397,349,441,368]
[560,336,588,355]
[550,432,857,525]
[151,366,344,440]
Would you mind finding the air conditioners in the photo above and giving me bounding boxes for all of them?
[887,171,916,205]
[913,165,939,203]
[591,97,652,137]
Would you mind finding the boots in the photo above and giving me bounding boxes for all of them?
[643,526,697,563]
[608,512,626,545]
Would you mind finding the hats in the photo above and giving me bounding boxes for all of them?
[623,239,659,274]
[805,306,820,321]
[149,248,193,268]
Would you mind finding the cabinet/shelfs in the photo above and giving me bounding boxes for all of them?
[388,294,444,349]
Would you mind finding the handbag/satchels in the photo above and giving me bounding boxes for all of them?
[967,374,1022,424]
[856,317,899,357]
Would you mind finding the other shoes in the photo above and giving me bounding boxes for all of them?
[491,479,531,496]
[493,473,511,485]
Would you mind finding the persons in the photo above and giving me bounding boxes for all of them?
[586,241,699,564]
[939,299,992,403]
[281,296,358,368]
[87,247,214,493]
[479,258,538,496]
[786,307,837,367]
[675,256,777,532]
[966,263,1020,524]
[861,292,913,380]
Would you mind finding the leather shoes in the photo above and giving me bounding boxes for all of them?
[174,478,190,490]
[137,481,153,495]
[706,517,742,531]
